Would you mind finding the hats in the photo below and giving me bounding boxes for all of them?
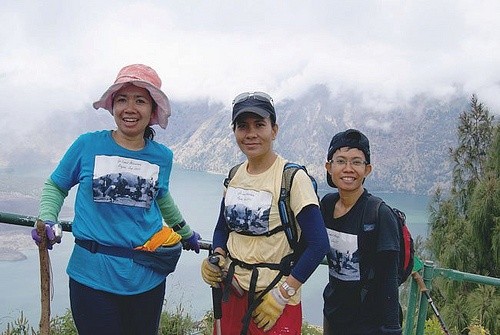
[93,64,171,129]
[326,129,371,188]
[231,96,276,124]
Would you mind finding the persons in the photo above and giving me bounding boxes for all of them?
[201,96,328,335]
[31,64,202,335]
[314,129,414,335]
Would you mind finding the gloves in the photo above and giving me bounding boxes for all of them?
[32,221,62,250]
[201,252,226,288]
[181,230,202,253]
[251,285,288,332]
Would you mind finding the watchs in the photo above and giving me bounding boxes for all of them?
[281,281,296,296]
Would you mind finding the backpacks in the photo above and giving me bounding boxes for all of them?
[321,193,414,285]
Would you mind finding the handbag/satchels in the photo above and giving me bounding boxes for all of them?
[74,225,182,275]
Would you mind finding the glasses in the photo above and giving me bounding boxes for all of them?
[332,159,368,168]
[232,91,274,110]
